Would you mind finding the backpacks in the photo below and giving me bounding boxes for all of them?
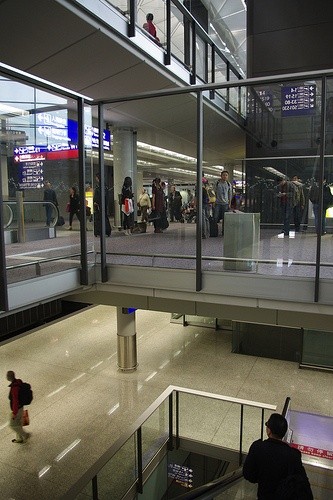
[19,382,33,406]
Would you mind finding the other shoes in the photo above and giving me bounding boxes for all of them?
[67,226,72,230]
[154,227,162,233]
[303,226,308,230]
[12,439,23,443]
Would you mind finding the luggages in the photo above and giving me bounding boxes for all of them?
[94,214,112,238]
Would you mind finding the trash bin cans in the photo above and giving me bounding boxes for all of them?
[224,211,260,271]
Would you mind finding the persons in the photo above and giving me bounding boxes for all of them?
[151,177,169,233]
[93,173,112,237]
[300,177,308,229]
[43,183,59,227]
[187,189,193,203]
[312,175,331,237]
[138,188,151,222]
[242,413,317,500]
[277,175,296,236]
[7,371,30,443]
[292,174,304,232]
[68,186,80,231]
[196,176,210,239]
[168,186,182,222]
[214,170,233,236]
[122,177,135,236]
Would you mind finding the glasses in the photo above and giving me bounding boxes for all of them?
[265,422,269,428]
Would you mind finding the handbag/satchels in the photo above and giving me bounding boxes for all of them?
[148,210,161,222]
[56,216,65,226]
[22,410,29,426]
[66,202,70,212]
[86,207,91,216]
